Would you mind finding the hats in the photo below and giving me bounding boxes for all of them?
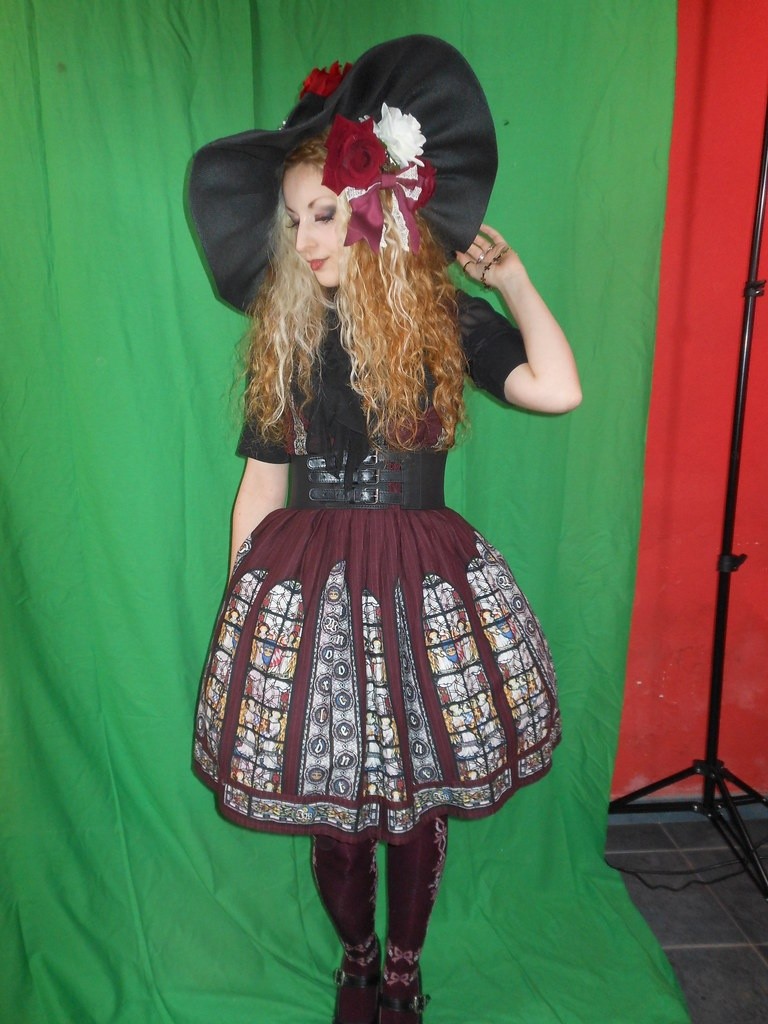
[187,34,497,320]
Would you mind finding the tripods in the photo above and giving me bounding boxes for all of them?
[603,115,768,898]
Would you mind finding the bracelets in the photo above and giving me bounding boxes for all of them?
[481,248,512,290]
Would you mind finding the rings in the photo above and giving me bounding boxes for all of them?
[462,244,495,272]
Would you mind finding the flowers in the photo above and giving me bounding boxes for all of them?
[301,59,353,101]
[319,103,439,250]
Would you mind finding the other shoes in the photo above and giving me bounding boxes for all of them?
[380,965,430,1024]
[335,934,380,1024]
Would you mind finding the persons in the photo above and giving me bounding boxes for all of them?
[186,34,582,1024]
